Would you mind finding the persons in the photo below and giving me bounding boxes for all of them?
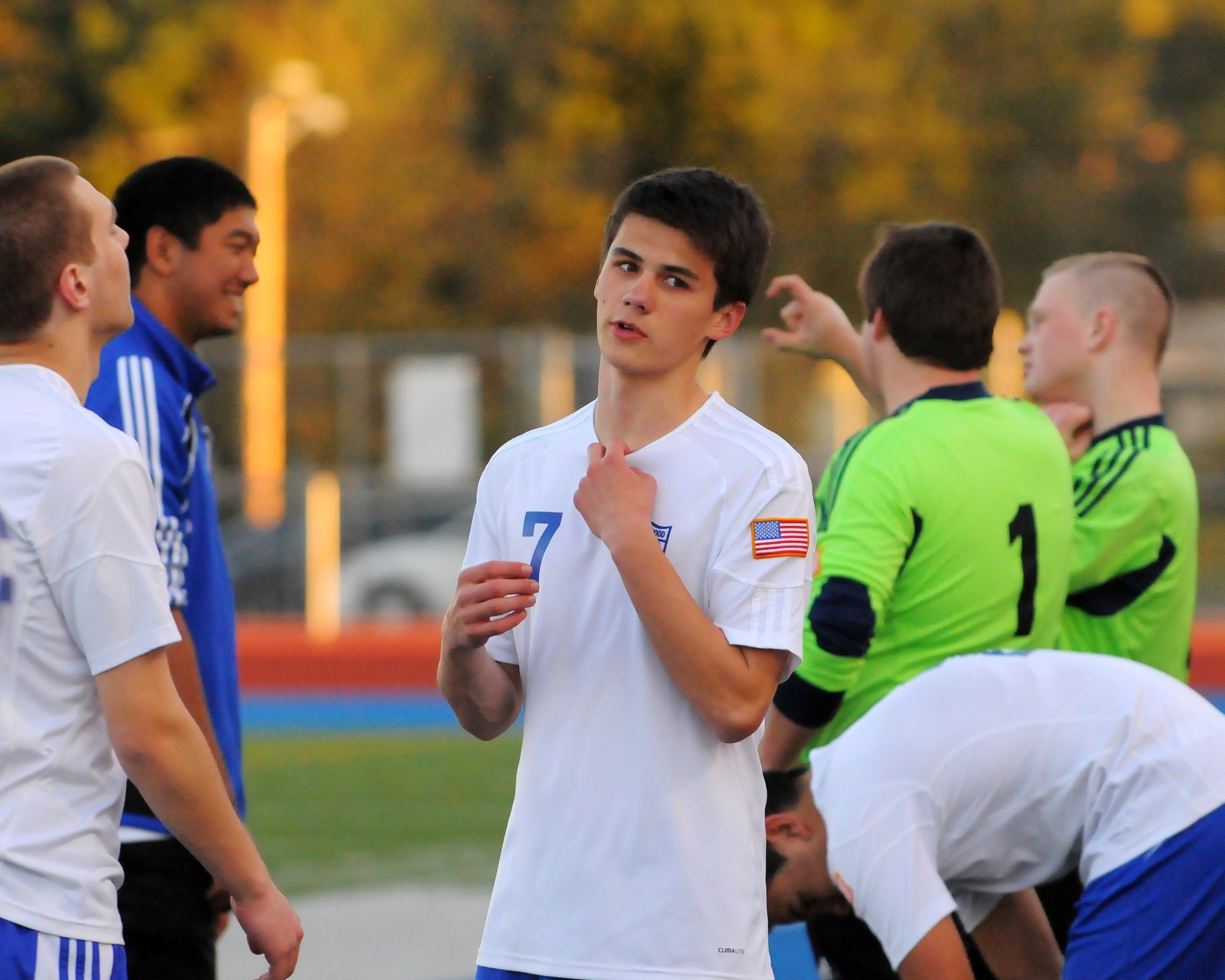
[0,155,304,979]
[438,167,817,980]
[761,645,1225,980]
[757,224,1073,980]
[84,157,260,980]
[1017,251,1199,963]
